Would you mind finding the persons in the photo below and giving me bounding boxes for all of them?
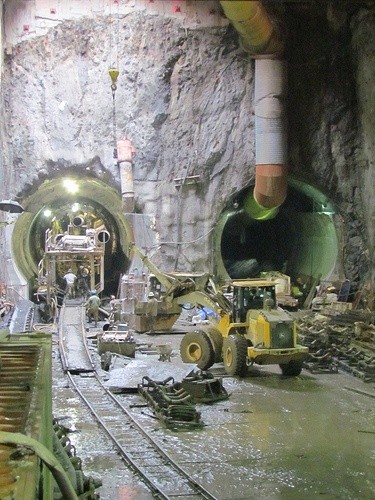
[146,292,157,335]
[247,289,262,306]
[80,265,89,291]
[110,295,127,322]
[63,268,77,299]
[85,290,100,325]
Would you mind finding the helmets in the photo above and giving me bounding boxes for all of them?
[91,290,96,295]
[68,269,72,272]
[79,266,84,270]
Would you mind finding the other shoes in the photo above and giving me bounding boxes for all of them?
[87,321,92,323]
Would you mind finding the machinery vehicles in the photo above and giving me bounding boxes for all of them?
[126,238,311,380]
